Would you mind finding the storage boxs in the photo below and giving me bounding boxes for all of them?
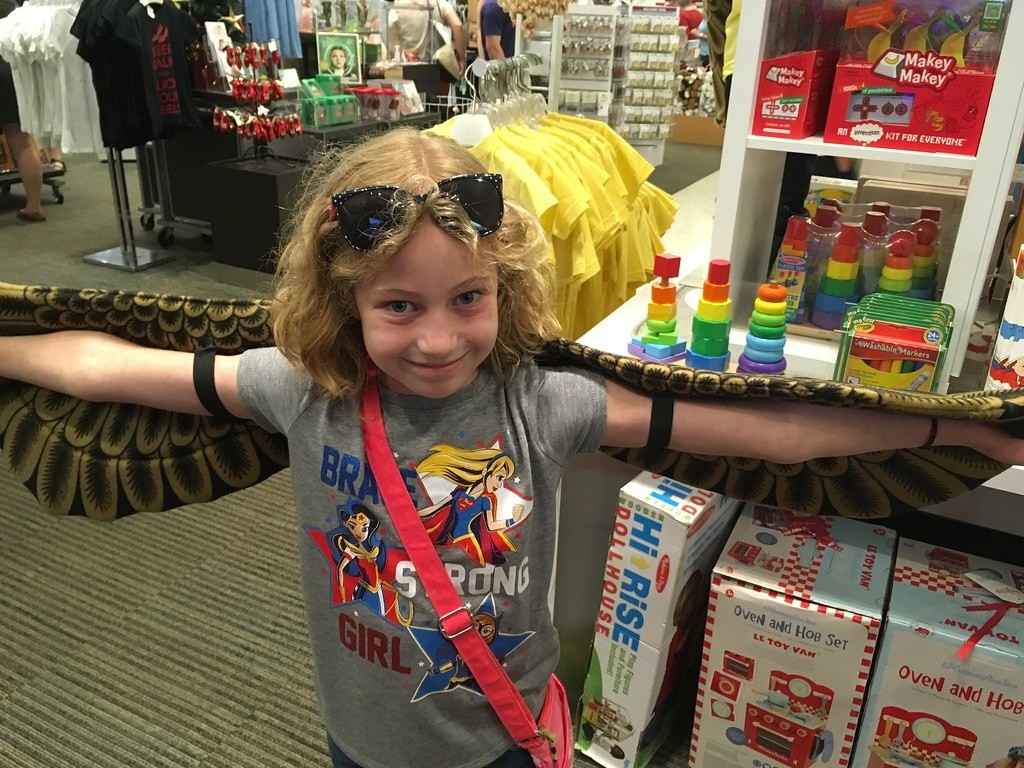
[571,469,746,768]
[849,537,1024,768]
[823,0,1013,158]
[688,502,897,768]
[752,46,840,140]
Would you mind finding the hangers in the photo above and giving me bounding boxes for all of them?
[139,0,163,6]
[447,52,551,149]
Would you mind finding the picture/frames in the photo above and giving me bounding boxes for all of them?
[316,32,362,85]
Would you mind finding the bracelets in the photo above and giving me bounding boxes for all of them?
[921,417,938,450]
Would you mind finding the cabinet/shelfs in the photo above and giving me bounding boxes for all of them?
[708,0,1024,395]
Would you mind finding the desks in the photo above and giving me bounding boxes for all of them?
[291,112,442,165]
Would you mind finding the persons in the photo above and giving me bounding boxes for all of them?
[679,0,742,129]
[386,0,467,111]
[480,0,515,91]
[0,124,1024,768]
[328,47,348,76]
[0,0,66,222]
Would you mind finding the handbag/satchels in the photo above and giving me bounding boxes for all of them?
[428,0,460,83]
[536,671,574,768]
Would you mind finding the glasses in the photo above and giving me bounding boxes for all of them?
[329,172,505,252]
